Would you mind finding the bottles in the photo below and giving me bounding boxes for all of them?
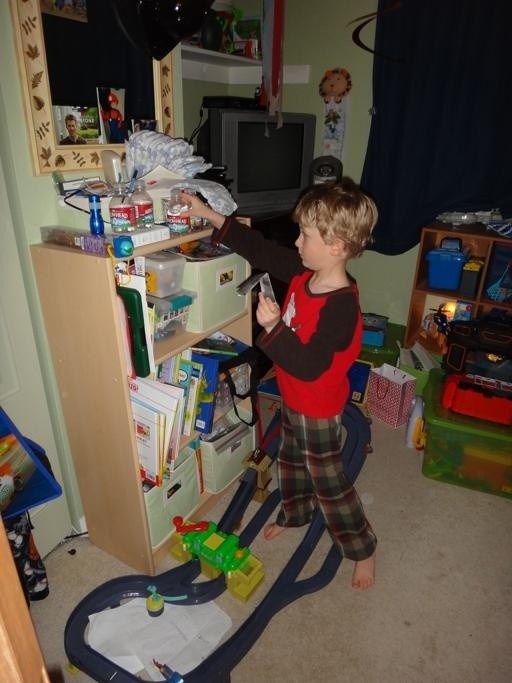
[166,188,209,234]
[109,179,155,234]
[400,393,426,449]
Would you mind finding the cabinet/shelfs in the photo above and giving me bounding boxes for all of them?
[30,204,267,576]
[402,227,512,358]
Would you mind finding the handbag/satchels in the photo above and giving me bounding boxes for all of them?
[366,363,417,429]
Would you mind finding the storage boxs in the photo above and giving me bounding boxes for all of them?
[423,367,512,499]
[142,404,253,549]
[426,247,473,291]
[144,247,250,342]
[359,312,408,368]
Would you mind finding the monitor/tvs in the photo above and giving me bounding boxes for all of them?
[196,108,315,214]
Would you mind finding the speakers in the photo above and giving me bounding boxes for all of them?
[311,156,343,187]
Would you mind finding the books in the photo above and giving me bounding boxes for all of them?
[110,273,250,487]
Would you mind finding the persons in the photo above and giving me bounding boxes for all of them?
[60,114,87,145]
[180,175,376,591]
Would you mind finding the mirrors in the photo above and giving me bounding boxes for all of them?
[8,0,174,175]
